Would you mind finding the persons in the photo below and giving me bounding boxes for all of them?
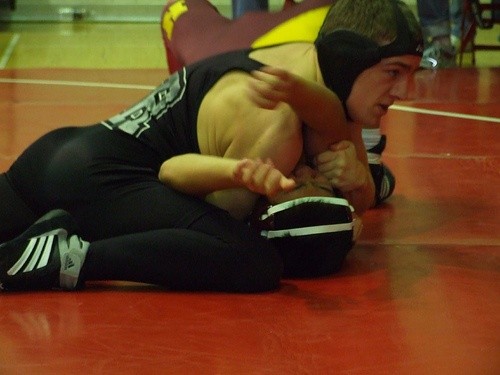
[159,1,395,279]
[0,0,426,296]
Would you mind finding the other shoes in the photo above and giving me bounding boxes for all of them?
[420,42,458,70]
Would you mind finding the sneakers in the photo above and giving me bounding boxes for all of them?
[0,207,89,289]
